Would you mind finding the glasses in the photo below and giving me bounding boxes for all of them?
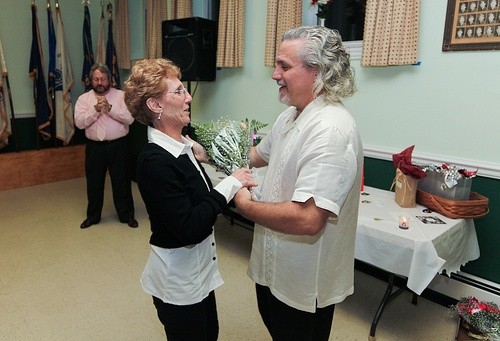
[167,87,188,98]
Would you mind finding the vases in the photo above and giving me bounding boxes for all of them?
[320,19,325,27]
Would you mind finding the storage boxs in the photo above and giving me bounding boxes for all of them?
[418,168,472,200]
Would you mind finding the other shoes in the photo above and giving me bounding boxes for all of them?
[127,219,138,227]
[79,219,92,228]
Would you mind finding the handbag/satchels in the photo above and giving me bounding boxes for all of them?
[394,168,417,208]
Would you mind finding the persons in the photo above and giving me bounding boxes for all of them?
[123,58,259,341]
[73,62,139,229]
[185,25,363,341]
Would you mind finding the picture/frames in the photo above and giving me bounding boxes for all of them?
[442,0,500,51]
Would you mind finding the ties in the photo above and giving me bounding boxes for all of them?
[97,112,105,141]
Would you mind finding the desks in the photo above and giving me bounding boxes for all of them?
[200,162,480,341]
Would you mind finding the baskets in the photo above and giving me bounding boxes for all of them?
[417,187,489,219]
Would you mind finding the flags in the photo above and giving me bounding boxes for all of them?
[81,0,122,92]
[45,0,77,146]
[29,0,55,150]
[0,31,16,153]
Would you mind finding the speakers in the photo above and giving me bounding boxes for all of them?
[162,16,218,82]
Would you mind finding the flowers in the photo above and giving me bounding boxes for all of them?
[190,119,270,194]
[449,296,500,341]
[311,0,333,19]
